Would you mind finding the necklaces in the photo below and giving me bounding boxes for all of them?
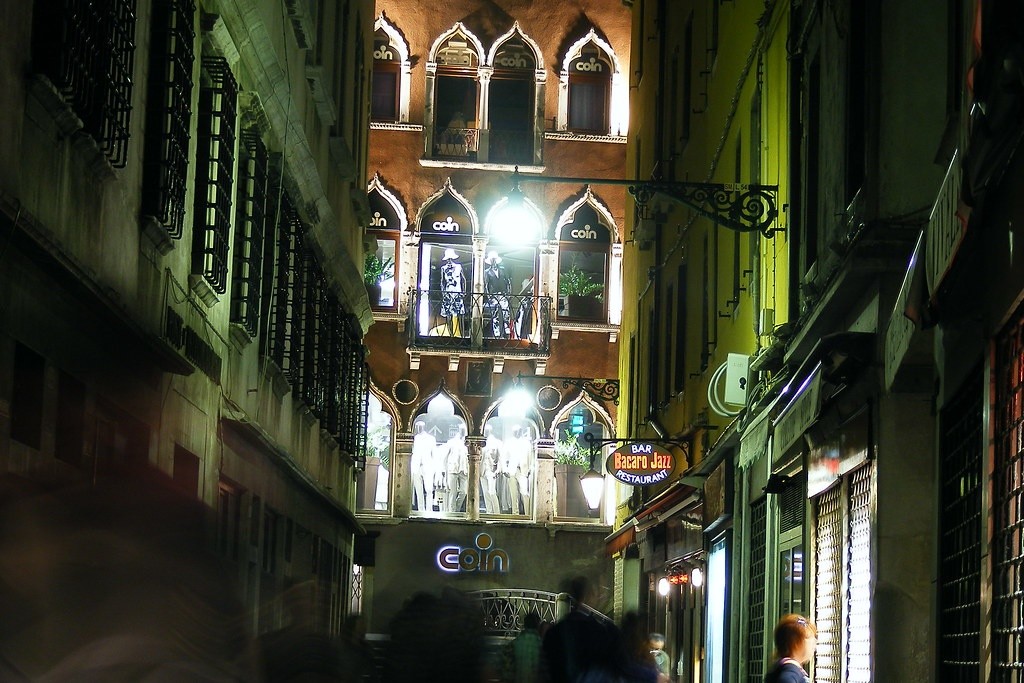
[498,613,543,682]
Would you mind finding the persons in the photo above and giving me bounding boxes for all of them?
[763,612,821,683]
[649,632,670,682]
[443,423,469,512]
[538,621,552,637]
[483,252,514,341]
[517,276,536,338]
[337,613,378,683]
[617,610,655,668]
[480,424,505,515]
[539,575,614,683]
[411,420,438,512]
[577,621,658,683]
[503,425,532,515]
[439,248,467,338]
